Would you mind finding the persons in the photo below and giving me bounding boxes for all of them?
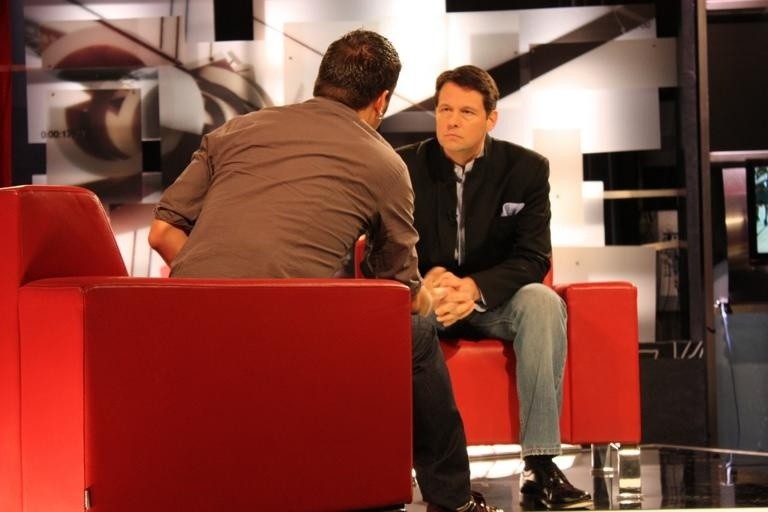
[146,25,492,511]
[357,64,591,509]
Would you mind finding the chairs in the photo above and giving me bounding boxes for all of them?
[0,185,413,511]
[354,233,642,510]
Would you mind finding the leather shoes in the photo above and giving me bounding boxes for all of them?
[517,464,591,510]
[425,490,504,512]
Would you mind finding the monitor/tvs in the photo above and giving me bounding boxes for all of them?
[746,159,768,268]
